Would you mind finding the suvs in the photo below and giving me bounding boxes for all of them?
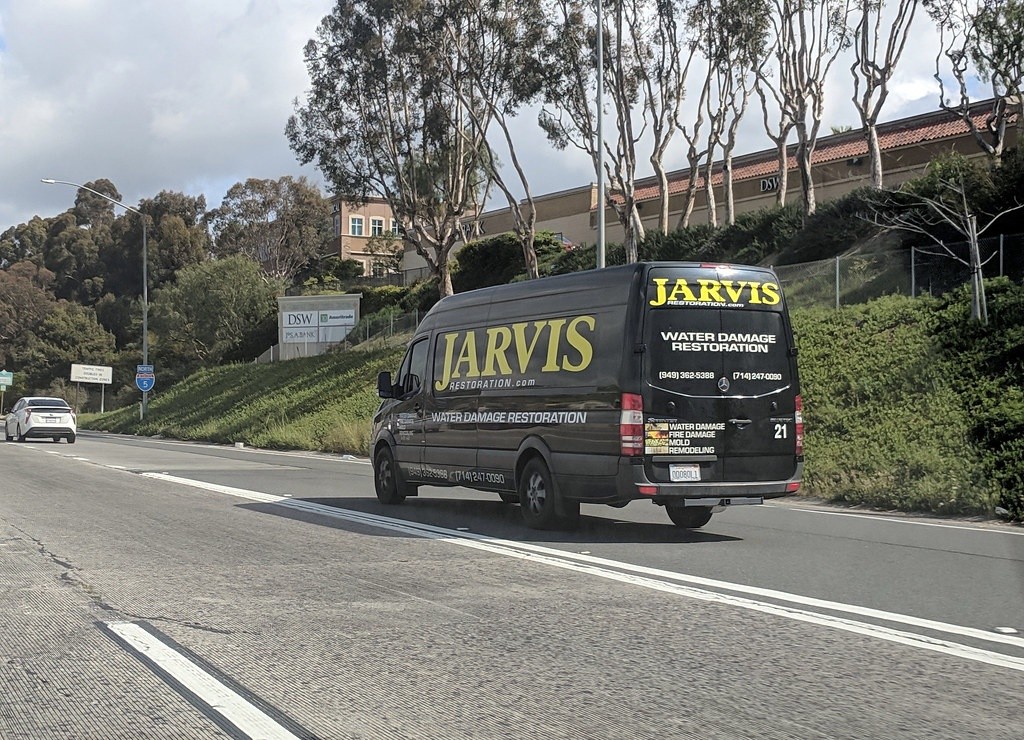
[3,396,77,444]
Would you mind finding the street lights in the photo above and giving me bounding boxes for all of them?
[40,176,149,422]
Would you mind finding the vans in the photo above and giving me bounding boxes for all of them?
[368,259,806,530]
